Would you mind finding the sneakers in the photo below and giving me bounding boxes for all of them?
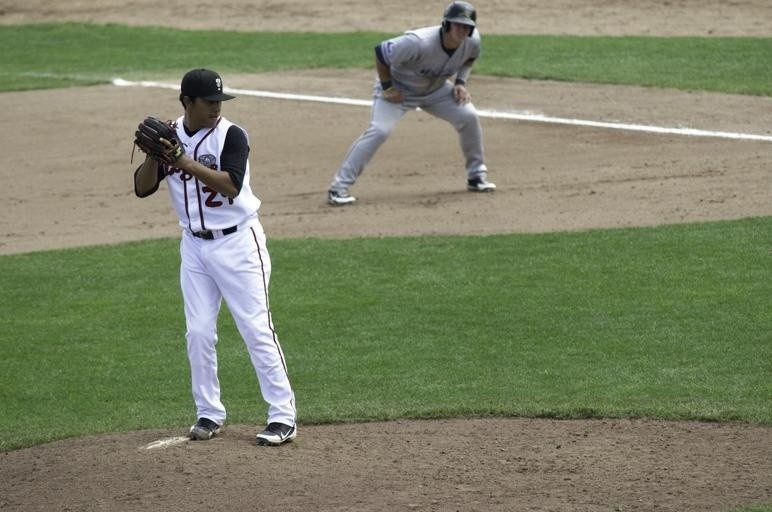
[467,177,497,191]
[256,422,297,446]
[188,418,223,440]
[328,189,356,205]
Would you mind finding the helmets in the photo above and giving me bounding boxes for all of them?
[441,0,477,37]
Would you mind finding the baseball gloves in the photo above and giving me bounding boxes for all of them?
[130,116,188,165]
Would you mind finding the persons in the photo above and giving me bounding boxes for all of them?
[326,0,499,207]
[129,68,298,447]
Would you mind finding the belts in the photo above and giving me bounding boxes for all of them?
[189,225,239,240]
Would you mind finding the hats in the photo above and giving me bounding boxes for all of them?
[179,67,236,102]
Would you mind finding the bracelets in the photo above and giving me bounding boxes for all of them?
[454,76,465,86]
[380,79,394,90]
